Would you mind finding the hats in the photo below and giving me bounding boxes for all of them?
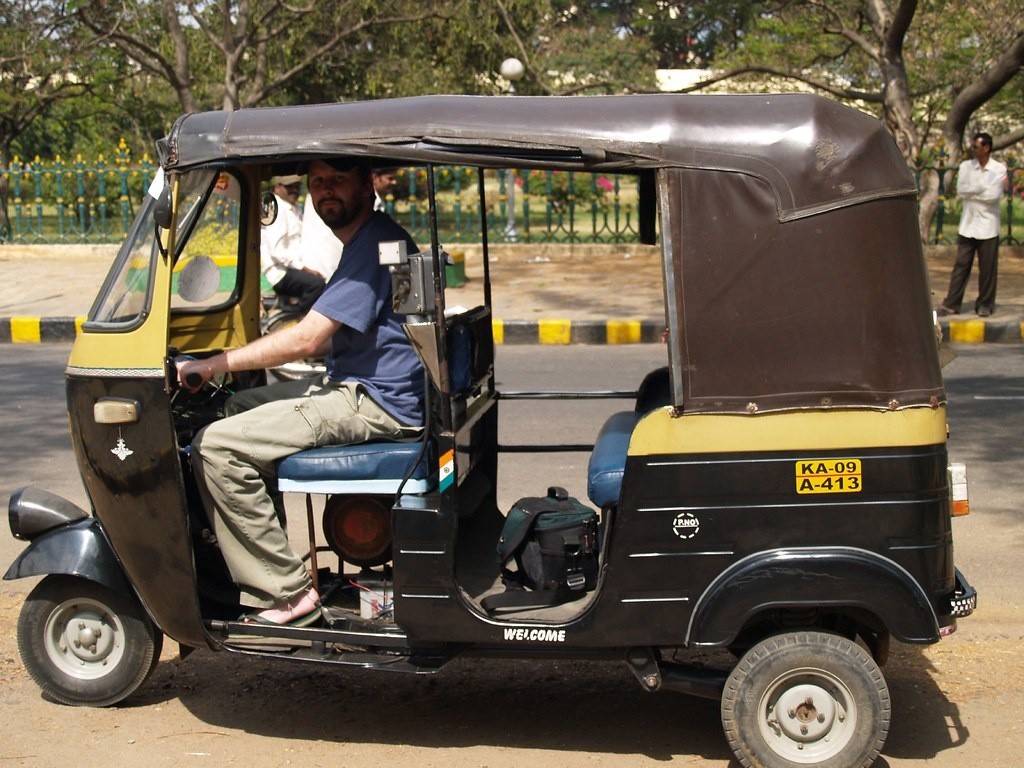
[271,174,303,187]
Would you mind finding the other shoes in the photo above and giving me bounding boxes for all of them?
[977,306,991,318]
[936,306,954,316]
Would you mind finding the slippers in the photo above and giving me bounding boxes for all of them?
[236,608,323,628]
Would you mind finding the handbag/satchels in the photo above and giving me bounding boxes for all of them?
[480,487,599,616]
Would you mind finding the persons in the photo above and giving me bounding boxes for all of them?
[937,133,1007,316]
[178,154,421,629]
[370,165,399,215]
[257,175,327,307]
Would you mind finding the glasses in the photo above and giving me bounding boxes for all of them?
[972,144,984,149]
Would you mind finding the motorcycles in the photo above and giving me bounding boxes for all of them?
[1,88,977,768]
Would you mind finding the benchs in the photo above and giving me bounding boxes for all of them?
[273,304,494,495]
[586,411,634,510]
